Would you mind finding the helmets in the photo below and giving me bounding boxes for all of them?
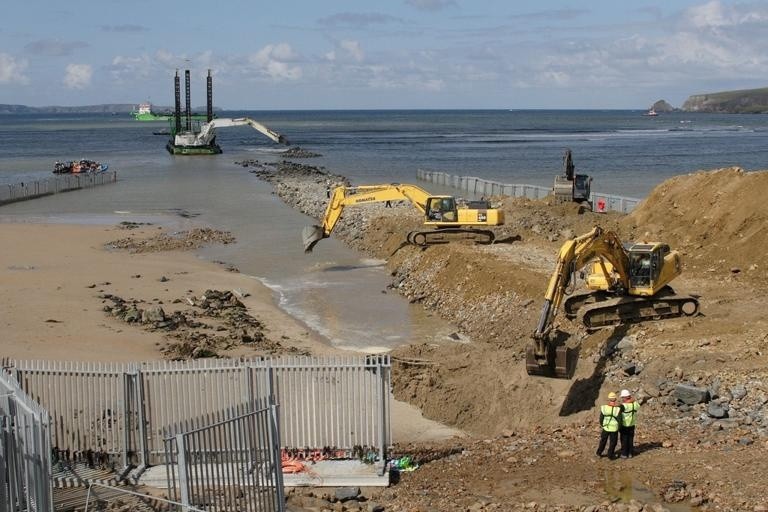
[619,389,631,398]
[608,392,617,398]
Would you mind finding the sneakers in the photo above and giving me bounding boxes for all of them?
[609,453,632,460]
[594,452,601,459]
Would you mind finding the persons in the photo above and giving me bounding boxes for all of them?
[385,200,392,208]
[620,390,640,458]
[327,186,330,198]
[637,256,650,285]
[595,392,621,460]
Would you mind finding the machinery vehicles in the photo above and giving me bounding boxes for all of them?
[552,149,594,215]
[302,182,505,255]
[164,115,290,156]
[523,222,703,379]
